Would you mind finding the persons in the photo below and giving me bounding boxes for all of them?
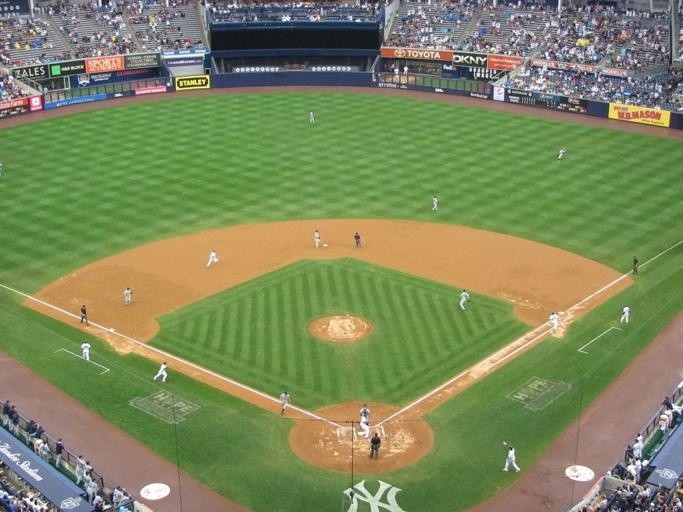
[357,404,371,438]
[621,305,630,324]
[549,312,559,332]
[557,148,564,160]
[459,289,470,311]
[124,287,132,304]
[0,400,136,512]
[353,232,362,248]
[369,432,381,459]
[501,445,520,473]
[632,256,638,274]
[314,230,320,249]
[432,196,440,211]
[81,340,90,362]
[1,1,206,119]
[575,395,683,512]
[309,111,316,125]
[207,0,389,23]
[207,249,220,268]
[280,392,291,415]
[154,361,167,382]
[388,0,682,118]
[80,305,89,325]
